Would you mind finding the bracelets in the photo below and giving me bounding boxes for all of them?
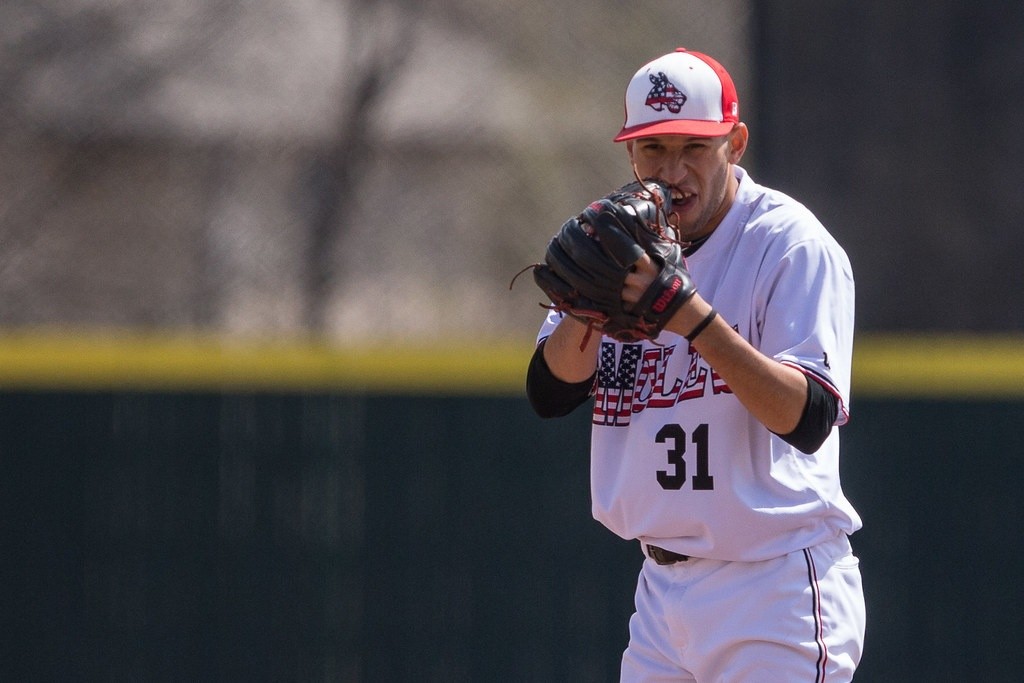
[684,308,718,342]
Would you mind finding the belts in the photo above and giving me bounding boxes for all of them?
[647,544,689,565]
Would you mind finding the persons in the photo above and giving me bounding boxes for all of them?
[526,47,867,683]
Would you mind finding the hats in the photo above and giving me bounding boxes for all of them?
[613,48,739,136]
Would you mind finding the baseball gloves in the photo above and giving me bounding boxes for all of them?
[529,178,696,342]
